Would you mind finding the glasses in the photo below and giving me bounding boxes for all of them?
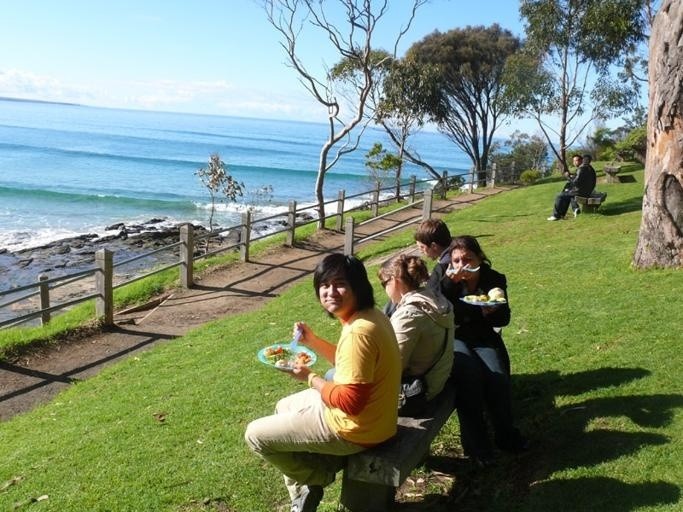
[381,279,390,288]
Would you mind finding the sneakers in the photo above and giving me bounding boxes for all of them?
[287,488,325,512]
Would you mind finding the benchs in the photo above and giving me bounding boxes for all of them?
[604,166,621,183]
[327,327,505,512]
[575,192,607,214]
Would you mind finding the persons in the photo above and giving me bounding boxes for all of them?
[374,252,456,410]
[382,217,454,308]
[547,153,594,222]
[561,155,595,217]
[244,253,405,512]
[435,238,513,473]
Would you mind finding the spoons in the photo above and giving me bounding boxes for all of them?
[286,328,304,352]
[448,266,481,273]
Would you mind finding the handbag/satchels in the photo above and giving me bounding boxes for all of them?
[398,375,428,417]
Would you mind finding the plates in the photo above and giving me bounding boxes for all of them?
[257,344,317,372]
[459,297,508,307]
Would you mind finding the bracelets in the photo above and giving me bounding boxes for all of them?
[306,373,317,388]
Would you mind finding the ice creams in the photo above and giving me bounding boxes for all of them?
[488,288,504,299]
